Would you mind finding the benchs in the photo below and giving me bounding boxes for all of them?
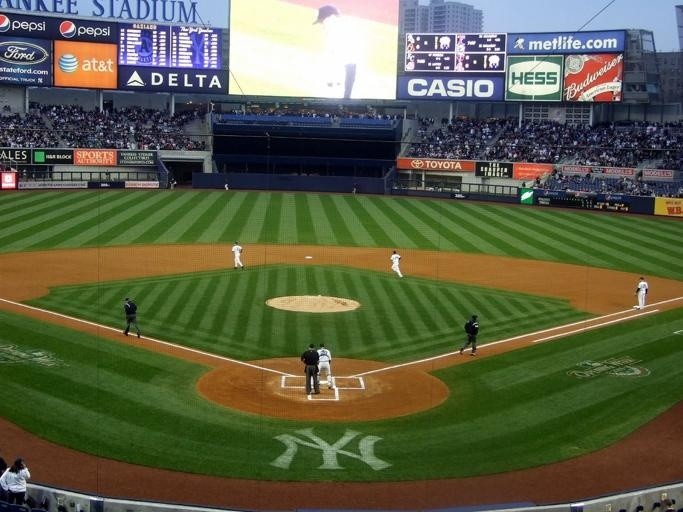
[0,499,48,512]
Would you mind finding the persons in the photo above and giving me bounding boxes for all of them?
[458,314,479,356]
[223,177,229,190]
[315,343,332,389]
[632,276,648,309]
[351,183,358,194]
[0,458,31,506]
[662,498,676,512]
[121,297,141,338]
[209,98,394,136]
[167,175,177,190]
[305,5,374,100]
[0,97,207,184]
[299,343,320,394]
[395,107,682,205]
[388,250,403,278]
[230,241,244,269]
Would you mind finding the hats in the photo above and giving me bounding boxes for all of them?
[312,6,337,24]
